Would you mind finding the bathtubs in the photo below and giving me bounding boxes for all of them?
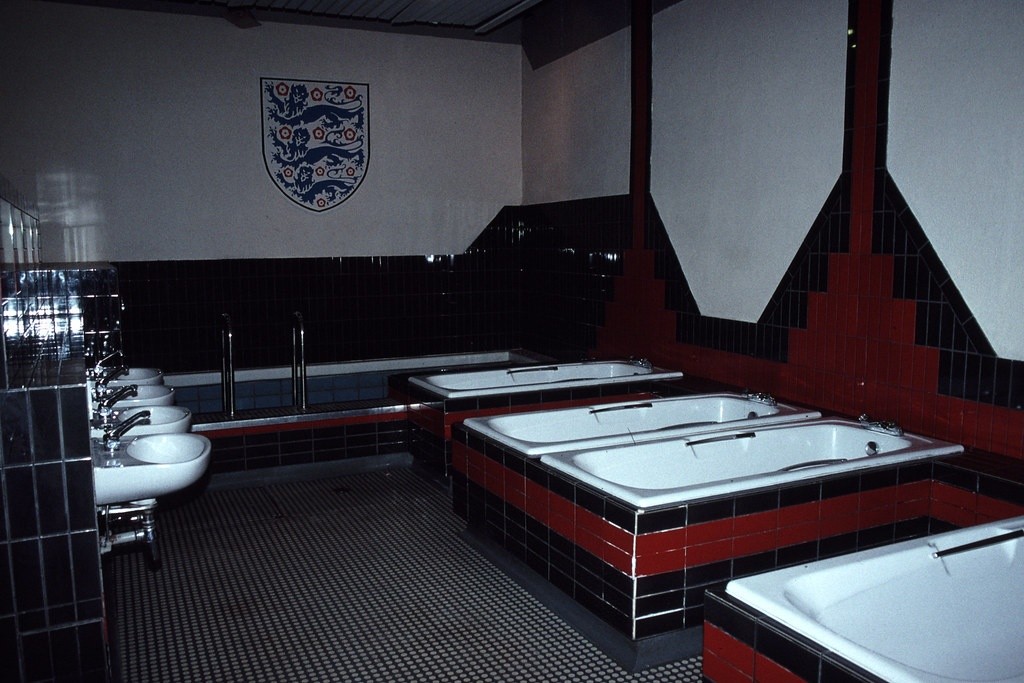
[703,510,1024,683]
[405,354,684,468]
[450,384,966,644]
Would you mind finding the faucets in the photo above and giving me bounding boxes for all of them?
[103,410,150,451]
[94,363,124,398]
[95,346,129,381]
[92,383,138,428]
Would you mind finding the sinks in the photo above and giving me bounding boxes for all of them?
[108,365,164,386]
[125,431,212,490]
[111,382,176,407]
[120,404,193,435]
